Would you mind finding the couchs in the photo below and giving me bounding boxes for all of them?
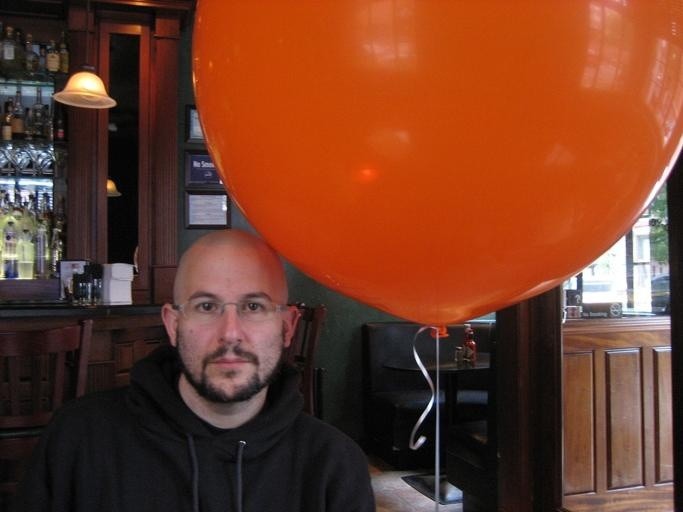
[360,319,497,512]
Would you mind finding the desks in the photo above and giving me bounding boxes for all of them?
[383,353,490,504]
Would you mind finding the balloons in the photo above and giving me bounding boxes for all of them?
[192,0,683,341]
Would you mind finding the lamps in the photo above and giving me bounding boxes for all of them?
[107,179,121,196]
[53,0,117,109]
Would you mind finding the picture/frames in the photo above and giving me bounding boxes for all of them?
[184,149,226,189]
[185,106,206,144]
[184,192,231,230]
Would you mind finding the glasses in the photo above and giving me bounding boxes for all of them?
[172,297,286,322]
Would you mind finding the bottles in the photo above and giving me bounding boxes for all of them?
[464,323,480,363]
[454,345,463,368]
[0,18,69,279]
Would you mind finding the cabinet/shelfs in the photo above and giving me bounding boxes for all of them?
[0,78,55,176]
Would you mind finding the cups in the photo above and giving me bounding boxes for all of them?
[63,278,104,307]
[566,306,583,318]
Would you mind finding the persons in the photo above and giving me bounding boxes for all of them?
[13,227,378,512]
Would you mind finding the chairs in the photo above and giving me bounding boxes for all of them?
[0,318,93,512]
[122,304,325,419]
